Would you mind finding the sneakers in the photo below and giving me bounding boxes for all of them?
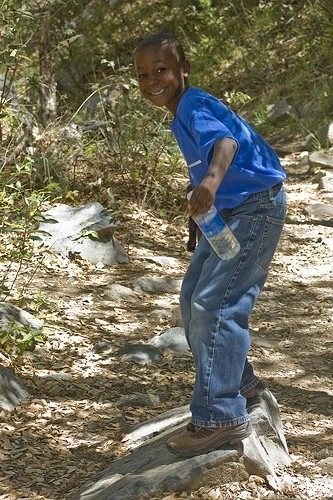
[243,379,267,399]
[167,421,254,457]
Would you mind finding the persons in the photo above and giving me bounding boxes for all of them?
[133,33,286,457]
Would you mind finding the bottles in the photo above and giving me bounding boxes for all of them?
[187,189,240,261]
